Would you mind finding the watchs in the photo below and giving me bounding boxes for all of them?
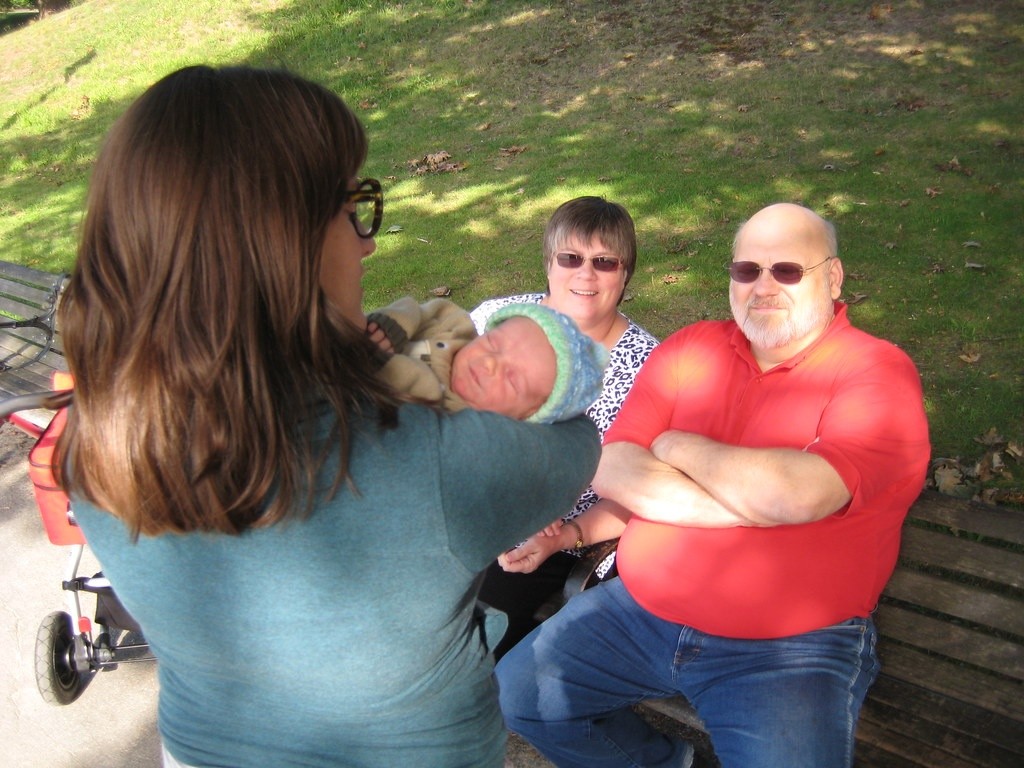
[565,520,584,550]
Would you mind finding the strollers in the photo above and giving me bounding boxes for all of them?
[0,370,161,711]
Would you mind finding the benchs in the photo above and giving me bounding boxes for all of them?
[0,258,1023,767]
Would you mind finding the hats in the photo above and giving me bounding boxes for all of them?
[484,303,609,424]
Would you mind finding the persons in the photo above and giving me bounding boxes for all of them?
[62,62,601,768]
[498,201,935,766]
[358,291,598,427]
[446,193,670,630]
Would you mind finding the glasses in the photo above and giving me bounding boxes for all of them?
[341,179,382,237]
[557,254,623,273]
[730,261,832,285]
